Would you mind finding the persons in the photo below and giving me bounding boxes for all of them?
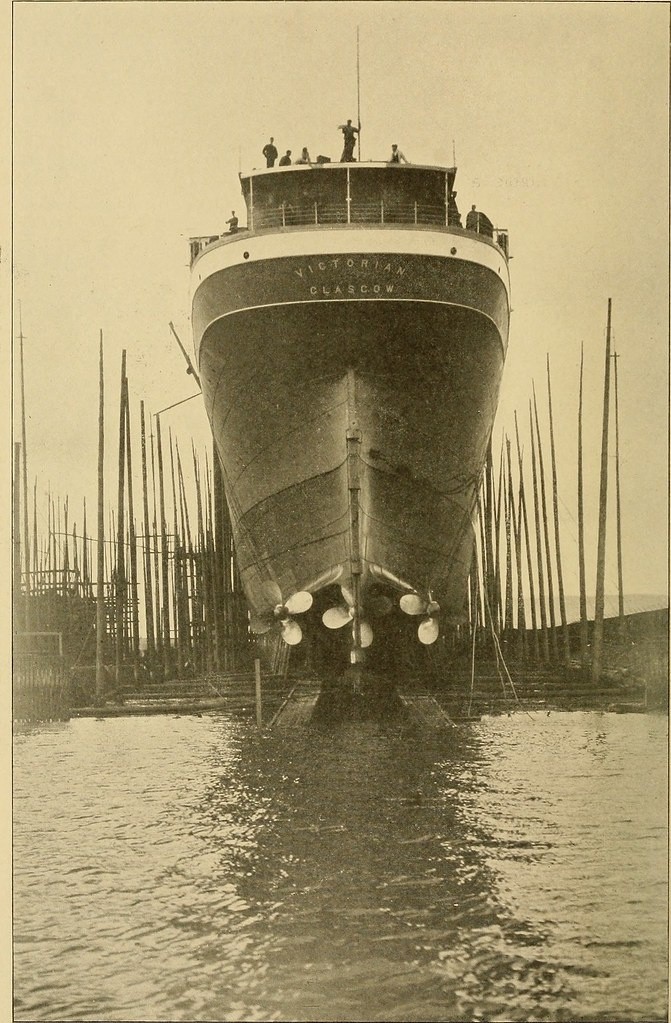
[226,211,238,231]
[279,150,292,166]
[262,137,278,168]
[389,144,408,164]
[339,120,361,162]
[302,147,310,163]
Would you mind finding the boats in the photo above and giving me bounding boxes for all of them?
[187,25,525,731]
